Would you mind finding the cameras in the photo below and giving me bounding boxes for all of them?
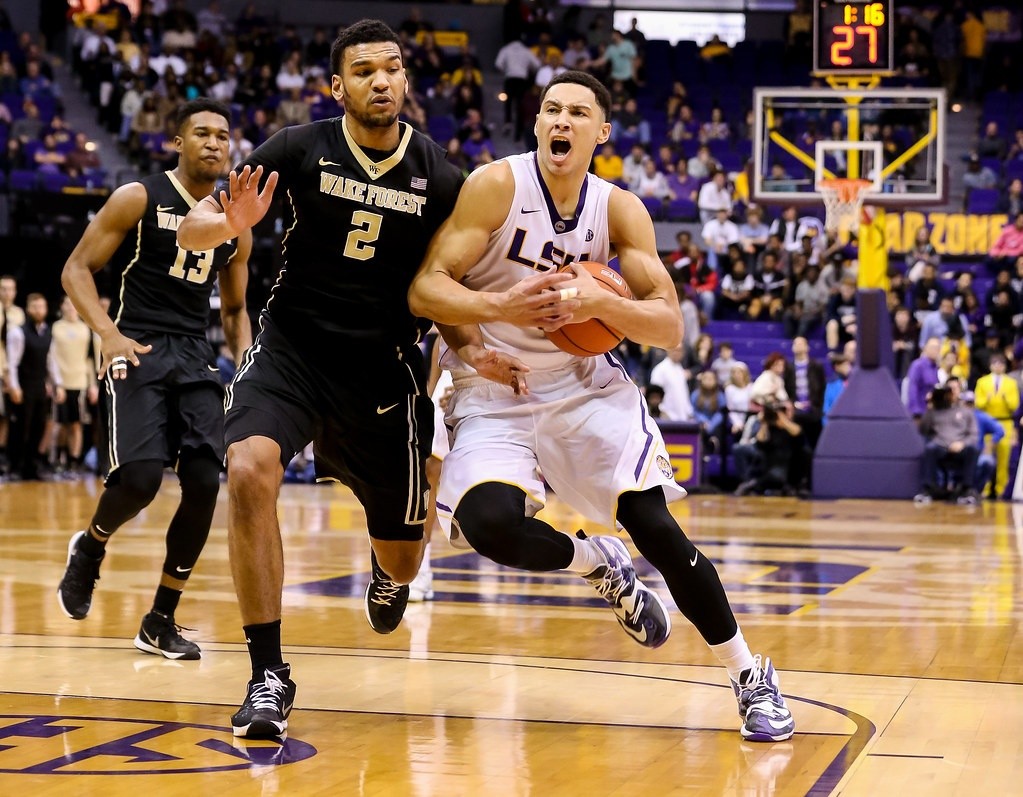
[762,404,785,421]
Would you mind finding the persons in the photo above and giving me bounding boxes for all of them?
[408,67,798,745]
[498,25,1023,511]
[1,2,494,482]
[408,330,548,605]
[175,21,467,737]
[56,98,253,662]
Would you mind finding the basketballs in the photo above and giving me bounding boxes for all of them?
[542,259,635,359]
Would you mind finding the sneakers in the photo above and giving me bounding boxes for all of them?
[729,655,795,740]
[231,663,296,736]
[579,534,672,648]
[362,546,412,633]
[406,570,435,601]
[134,615,203,660]
[741,745,791,780]
[56,530,106,619]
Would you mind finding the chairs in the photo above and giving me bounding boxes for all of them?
[0,1,1023,493]
